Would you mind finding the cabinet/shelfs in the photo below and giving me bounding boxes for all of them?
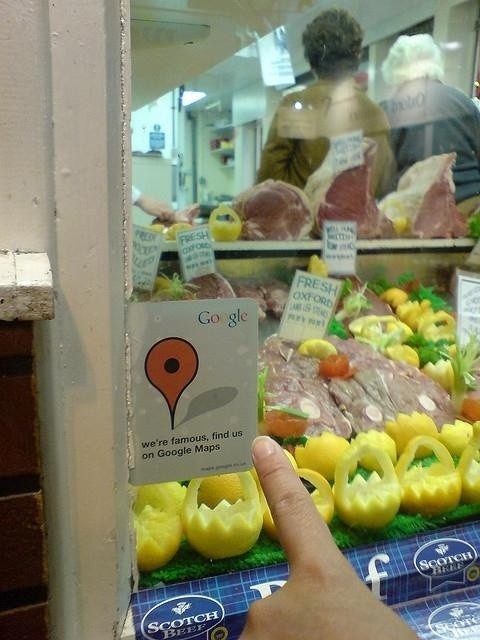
[198,97,239,206]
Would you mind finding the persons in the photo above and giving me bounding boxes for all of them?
[239,434,417,639]
[131,185,175,222]
[252,8,397,206]
[380,32,480,219]
